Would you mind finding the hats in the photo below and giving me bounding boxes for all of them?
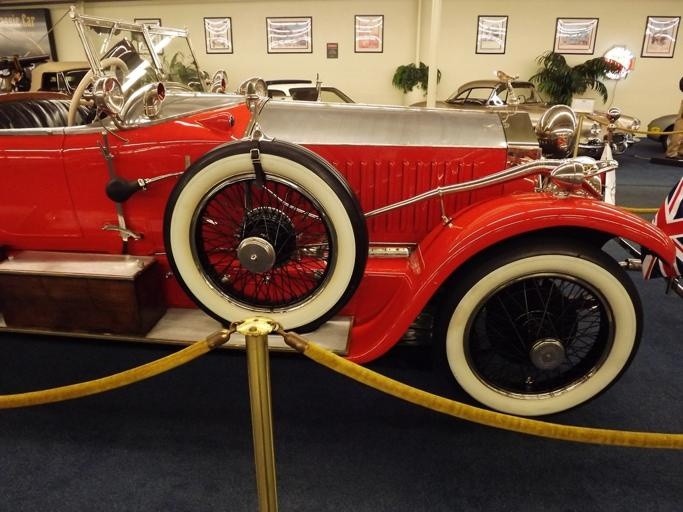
[645,113,678,147]
[401,76,642,158]
[243,76,361,105]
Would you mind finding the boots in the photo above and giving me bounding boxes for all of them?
[0,4,678,421]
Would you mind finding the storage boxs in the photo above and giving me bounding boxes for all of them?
[1,250,168,337]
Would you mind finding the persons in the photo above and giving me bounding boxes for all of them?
[10,69,30,92]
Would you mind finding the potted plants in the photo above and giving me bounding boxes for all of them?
[133,18,164,56]
[354,15,384,53]
[640,16,681,58]
[201,16,233,56]
[266,16,312,54]
[476,15,509,54]
[553,17,599,55]
